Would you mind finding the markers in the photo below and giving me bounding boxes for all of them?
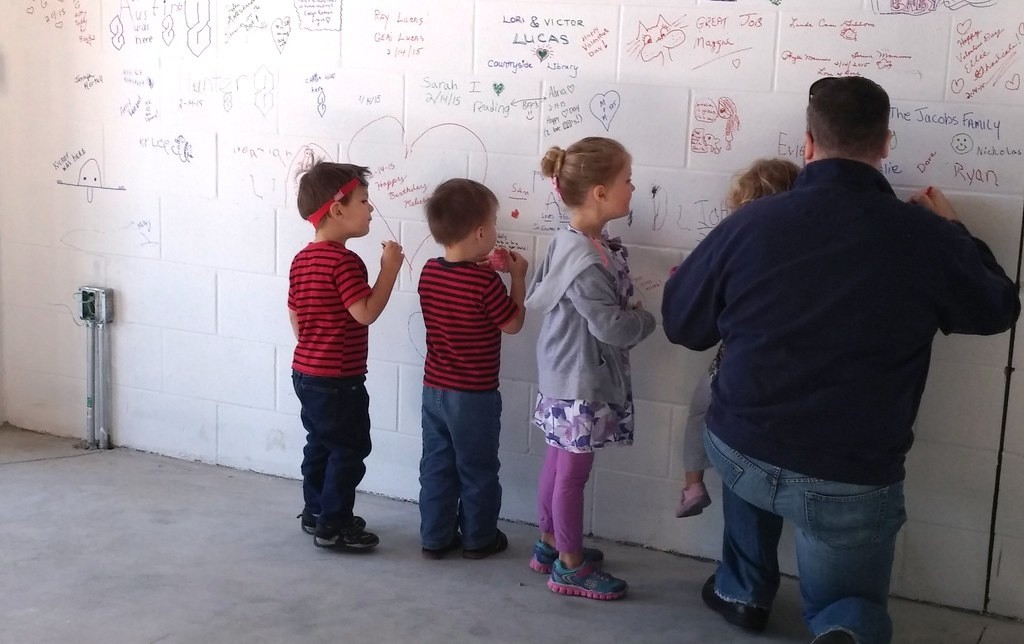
[380,242,386,247]
[926,186,933,197]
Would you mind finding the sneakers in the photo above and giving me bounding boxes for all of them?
[313,525,380,552]
[296,509,366,535]
[546,557,628,600]
[529,538,604,573]
[676,482,712,518]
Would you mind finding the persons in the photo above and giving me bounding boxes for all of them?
[287,153,405,550]
[417,178,529,561]
[661,75,1021,644]
[670,158,804,518]
[524,136,656,601]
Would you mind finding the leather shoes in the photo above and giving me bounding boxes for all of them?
[702,573,768,634]
[814,630,855,644]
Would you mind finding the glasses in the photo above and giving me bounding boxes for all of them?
[807,77,882,145]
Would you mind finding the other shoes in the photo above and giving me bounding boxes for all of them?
[462,527,508,559]
[421,531,461,559]
